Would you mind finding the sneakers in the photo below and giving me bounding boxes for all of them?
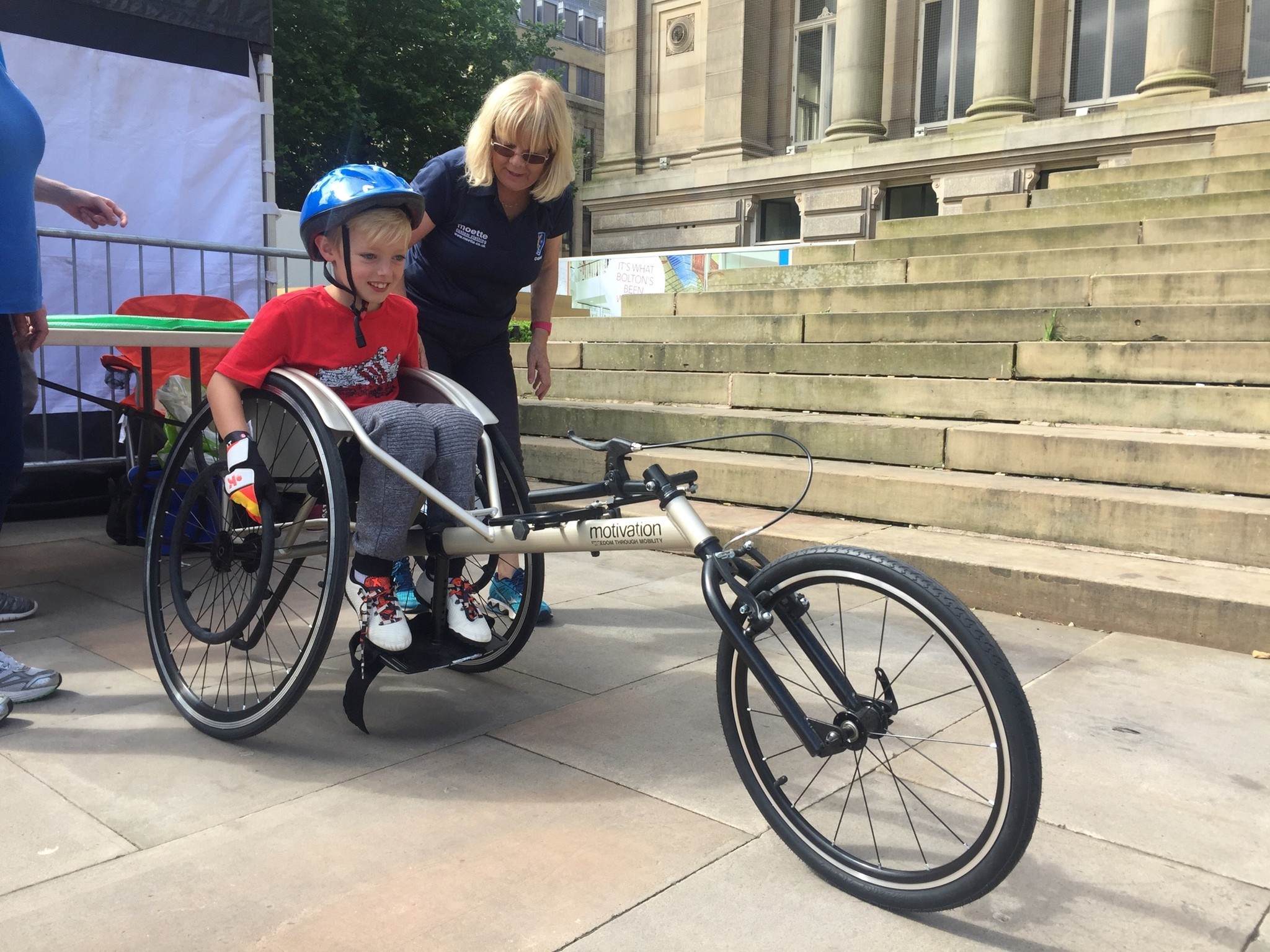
[0,592,37,621]
[415,573,493,645]
[392,557,420,611]
[487,567,553,623]
[0,693,14,721]
[349,565,412,651]
[0,650,62,703]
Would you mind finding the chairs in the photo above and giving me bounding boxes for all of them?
[103,293,251,532]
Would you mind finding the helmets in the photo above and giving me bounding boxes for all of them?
[298,162,426,262]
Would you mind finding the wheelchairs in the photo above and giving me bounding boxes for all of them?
[143,363,1043,911]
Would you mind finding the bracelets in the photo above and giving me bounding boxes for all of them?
[530,321,552,336]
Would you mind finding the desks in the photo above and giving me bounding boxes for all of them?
[25,313,257,546]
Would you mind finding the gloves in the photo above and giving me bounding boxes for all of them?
[226,438,284,525]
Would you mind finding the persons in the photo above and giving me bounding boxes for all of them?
[0,36,63,730]
[388,69,575,627]
[205,164,497,654]
[6,173,128,423]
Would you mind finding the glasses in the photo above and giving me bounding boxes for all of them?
[490,137,550,165]
